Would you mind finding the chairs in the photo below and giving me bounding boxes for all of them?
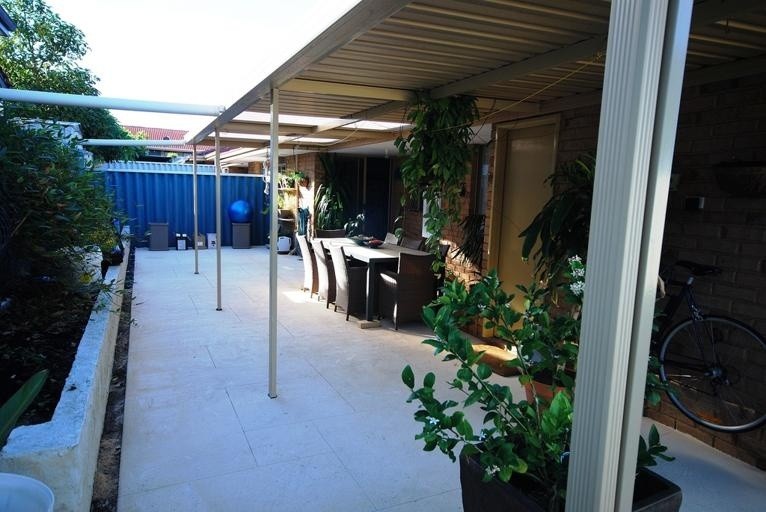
[295,228,450,331]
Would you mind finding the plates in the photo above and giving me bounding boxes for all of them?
[363,240,383,249]
[350,236,374,246]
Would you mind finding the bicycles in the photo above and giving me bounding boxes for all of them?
[651,260,766,433]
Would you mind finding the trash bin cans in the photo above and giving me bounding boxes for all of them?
[232,222,252,249]
[148,222,169,251]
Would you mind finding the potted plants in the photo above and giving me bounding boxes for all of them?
[401,150,684,512]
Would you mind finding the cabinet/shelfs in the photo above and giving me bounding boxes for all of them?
[277,188,296,222]
